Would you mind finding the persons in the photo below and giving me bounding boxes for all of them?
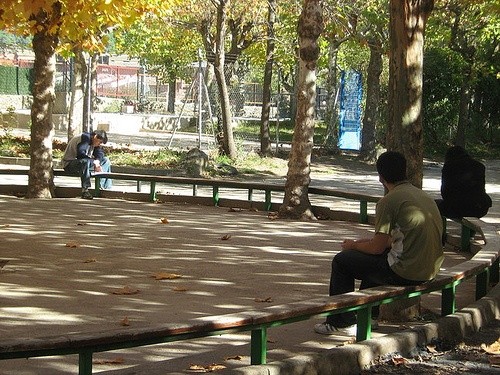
[434,146,492,247]
[314,152,444,336]
[61,130,112,199]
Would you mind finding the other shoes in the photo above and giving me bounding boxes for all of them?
[81,191,93,199]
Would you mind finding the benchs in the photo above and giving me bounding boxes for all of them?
[0,170,500,375]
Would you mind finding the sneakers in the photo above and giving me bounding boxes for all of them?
[313,322,357,337]
